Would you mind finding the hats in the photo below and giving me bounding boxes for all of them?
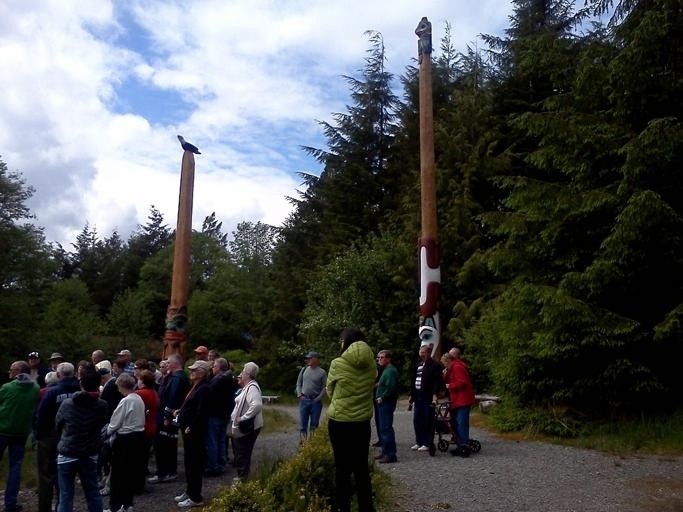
[28,352,40,360]
[187,360,210,371]
[117,350,131,355]
[48,353,64,360]
[195,346,208,352]
[304,352,319,357]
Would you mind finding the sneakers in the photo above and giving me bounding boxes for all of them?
[418,445,429,451]
[95,475,111,495]
[411,444,420,450]
[374,454,384,459]
[103,505,135,512]
[177,498,204,507]
[148,476,158,482]
[372,441,382,447]
[160,474,180,481]
[379,456,397,462]
[175,493,188,502]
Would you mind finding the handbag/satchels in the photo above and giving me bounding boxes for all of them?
[239,416,254,433]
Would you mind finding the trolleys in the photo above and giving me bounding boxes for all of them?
[430,396,483,454]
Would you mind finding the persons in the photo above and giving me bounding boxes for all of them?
[408,346,441,451]
[373,349,385,447]
[374,351,398,463]
[442,347,460,444]
[1,344,263,512]
[296,351,327,441]
[440,351,474,456]
[326,326,377,512]
